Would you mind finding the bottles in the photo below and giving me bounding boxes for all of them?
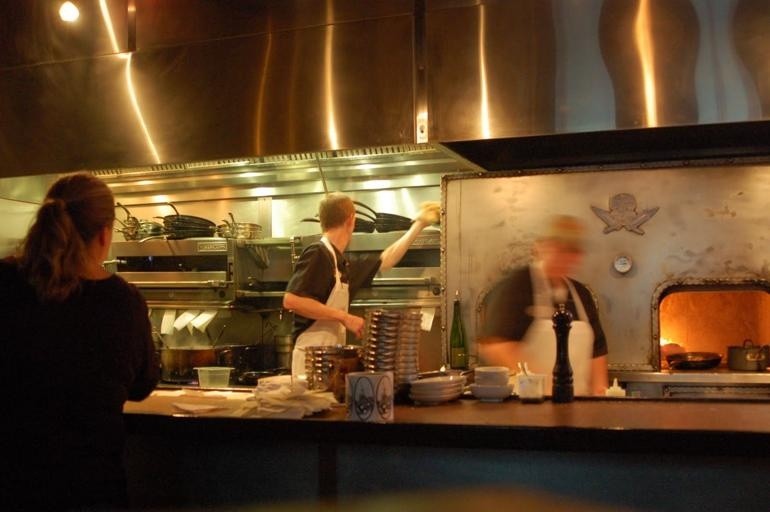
[606,379,626,398]
[448,297,467,370]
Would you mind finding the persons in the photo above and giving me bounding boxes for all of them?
[281,188,441,377]
[473,213,610,396]
[0,174,158,512]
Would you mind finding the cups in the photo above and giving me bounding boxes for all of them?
[519,373,546,403]
[346,373,394,423]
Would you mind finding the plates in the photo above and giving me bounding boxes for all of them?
[303,308,465,403]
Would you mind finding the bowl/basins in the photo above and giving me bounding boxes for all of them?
[244,370,274,383]
[470,367,515,403]
[193,367,235,389]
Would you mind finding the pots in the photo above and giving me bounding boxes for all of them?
[113,202,263,244]
[301,199,416,233]
[666,338,767,371]
[240,277,289,293]
[273,335,298,370]
[160,344,256,383]
